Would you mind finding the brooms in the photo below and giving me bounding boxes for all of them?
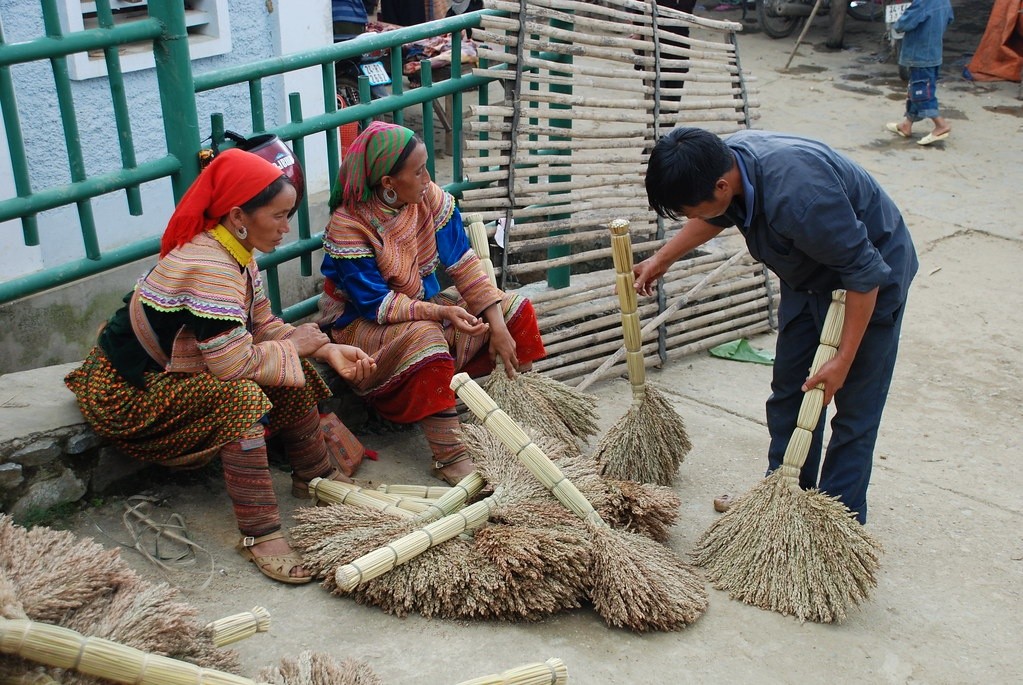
[684,287,886,628]
[0,211,710,685]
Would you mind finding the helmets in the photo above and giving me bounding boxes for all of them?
[201,130,304,219]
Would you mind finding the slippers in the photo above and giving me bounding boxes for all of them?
[916,131,948,145]
[887,122,907,138]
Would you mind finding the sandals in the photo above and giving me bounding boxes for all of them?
[291,467,364,499]
[432,456,494,497]
[235,530,312,583]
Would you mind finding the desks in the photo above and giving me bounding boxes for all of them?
[413,57,506,157]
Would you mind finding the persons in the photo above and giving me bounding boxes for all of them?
[331,0,484,88]
[613,127,919,532]
[312,120,547,488]
[885,0,955,144]
[63,148,377,584]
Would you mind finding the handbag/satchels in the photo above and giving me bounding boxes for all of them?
[336,95,357,161]
[318,412,378,477]
